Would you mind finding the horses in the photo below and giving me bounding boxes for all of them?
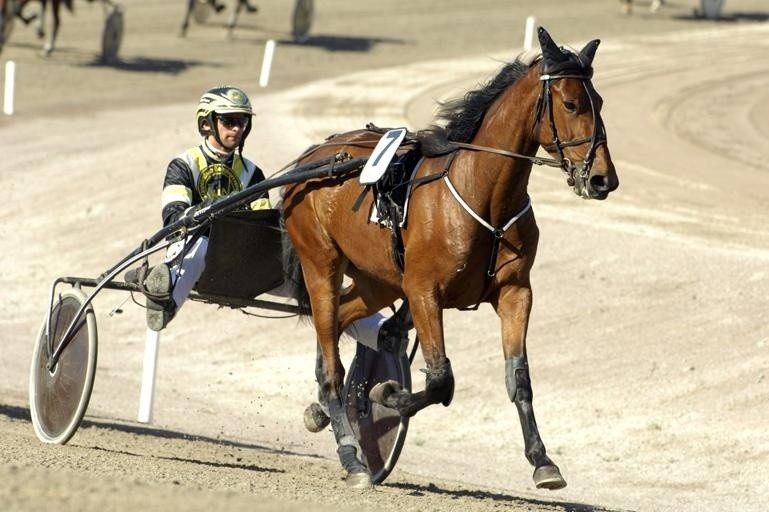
[277,26,620,491]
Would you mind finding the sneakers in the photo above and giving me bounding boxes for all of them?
[144,263,176,331]
[378,296,415,359]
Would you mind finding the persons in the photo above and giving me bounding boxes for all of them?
[145,85,410,359]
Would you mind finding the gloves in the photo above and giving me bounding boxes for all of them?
[201,195,235,216]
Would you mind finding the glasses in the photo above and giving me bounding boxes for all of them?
[216,116,250,129]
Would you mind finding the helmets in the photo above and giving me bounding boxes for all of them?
[196,86,256,137]
